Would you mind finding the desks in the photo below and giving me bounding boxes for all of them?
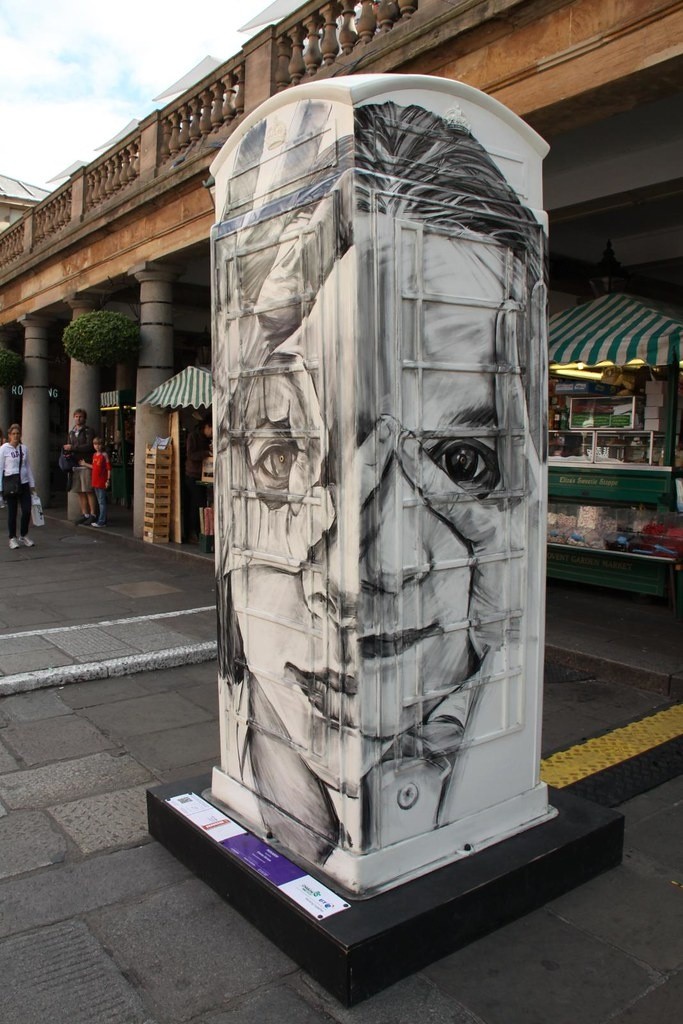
[196,480,214,507]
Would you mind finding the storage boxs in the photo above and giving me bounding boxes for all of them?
[198,532,215,553]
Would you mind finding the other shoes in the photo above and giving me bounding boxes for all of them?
[75,514,90,524]
[83,514,98,525]
[93,524,106,528]
[91,522,96,526]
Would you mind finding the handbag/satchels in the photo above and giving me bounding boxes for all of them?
[2,474,21,493]
[59,448,74,471]
[31,491,44,526]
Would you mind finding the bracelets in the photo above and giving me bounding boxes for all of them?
[106,479,110,484]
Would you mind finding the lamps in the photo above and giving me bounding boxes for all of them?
[549,359,647,381]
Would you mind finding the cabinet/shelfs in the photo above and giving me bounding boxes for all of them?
[142,443,172,543]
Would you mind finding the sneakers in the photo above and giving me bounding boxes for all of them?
[19,535,35,547]
[8,537,20,549]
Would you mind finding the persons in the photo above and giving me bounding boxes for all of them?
[184,414,212,545]
[0,423,39,549]
[78,437,112,528]
[62,408,98,526]
